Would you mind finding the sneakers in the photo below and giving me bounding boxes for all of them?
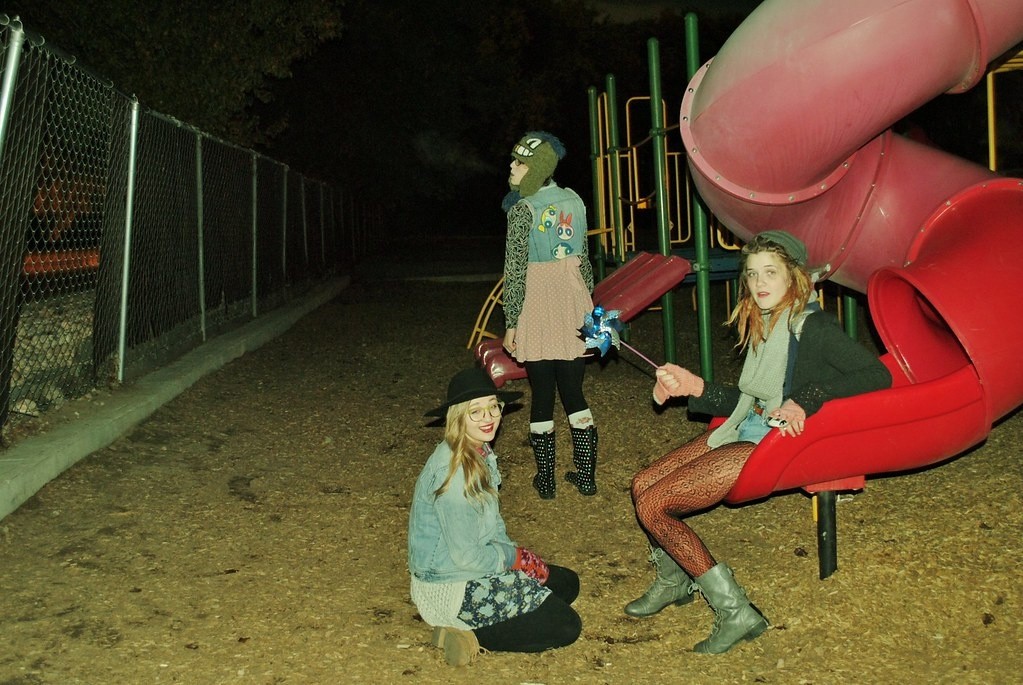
[432,625,490,667]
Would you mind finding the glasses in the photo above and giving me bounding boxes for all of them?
[465,402,506,422]
[511,156,525,166]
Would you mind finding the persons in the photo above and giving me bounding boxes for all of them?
[624,230,894,652]
[500,130,598,498]
[407,368,581,666]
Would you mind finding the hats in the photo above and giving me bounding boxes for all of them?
[755,230,806,267]
[509,131,566,198]
[423,368,520,417]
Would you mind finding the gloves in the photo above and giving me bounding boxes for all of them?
[511,547,548,585]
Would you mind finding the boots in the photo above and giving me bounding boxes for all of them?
[528,428,557,500]
[688,562,769,657]
[563,426,599,495]
[623,542,695,616]
